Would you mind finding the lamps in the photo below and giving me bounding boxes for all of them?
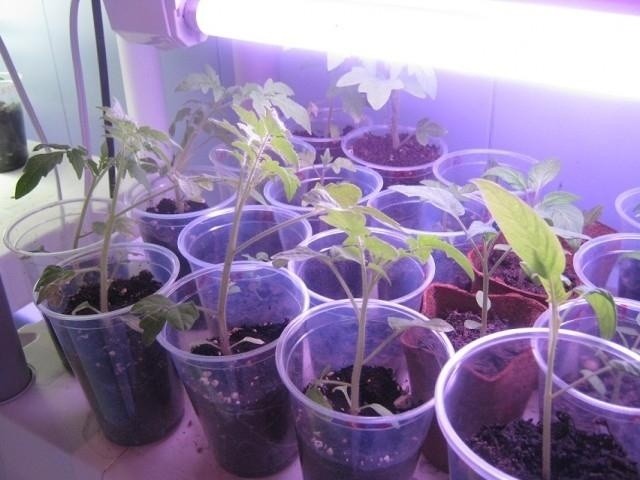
[103,0,640,100]
